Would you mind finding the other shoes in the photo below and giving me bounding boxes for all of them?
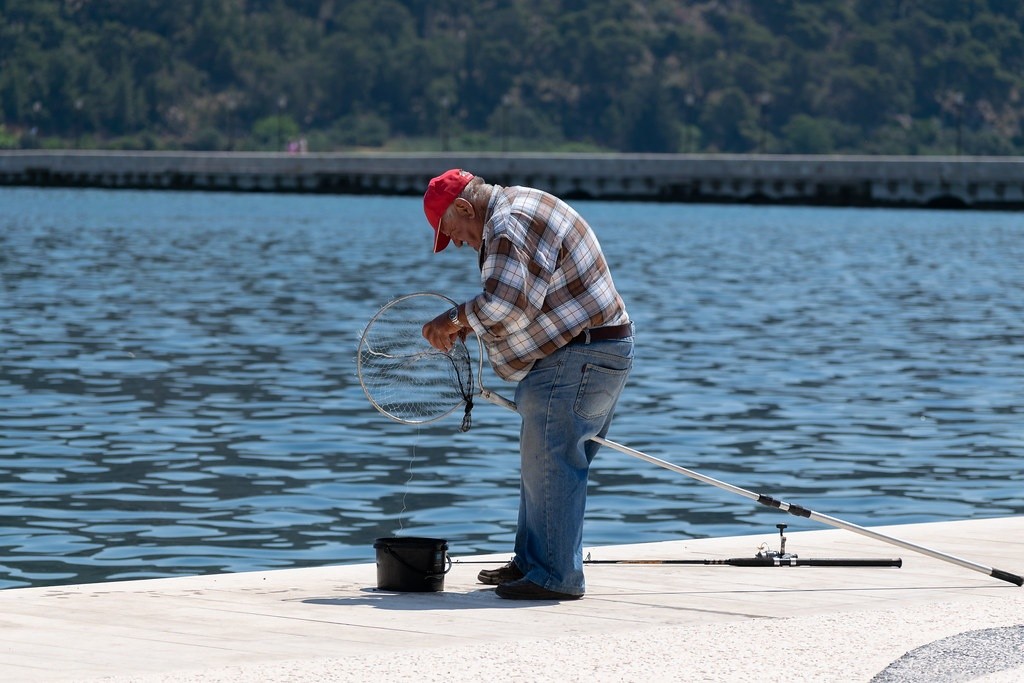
[478,559,523,585]
[496,575,585,600]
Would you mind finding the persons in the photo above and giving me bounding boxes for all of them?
[421,169,635,601]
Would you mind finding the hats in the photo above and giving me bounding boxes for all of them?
[423,168,474,254]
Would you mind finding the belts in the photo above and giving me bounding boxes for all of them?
[574,322,632,342]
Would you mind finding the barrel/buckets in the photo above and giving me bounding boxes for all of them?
[372,536,452,593]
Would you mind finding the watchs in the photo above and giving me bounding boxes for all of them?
[449,307,464,327]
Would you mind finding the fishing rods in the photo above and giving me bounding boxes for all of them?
[448,521,905,570]
